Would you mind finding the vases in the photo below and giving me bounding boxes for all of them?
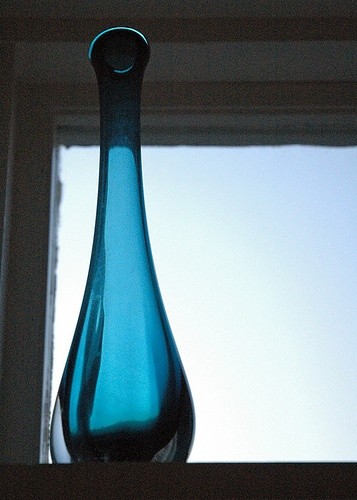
[48,26,195,465]
